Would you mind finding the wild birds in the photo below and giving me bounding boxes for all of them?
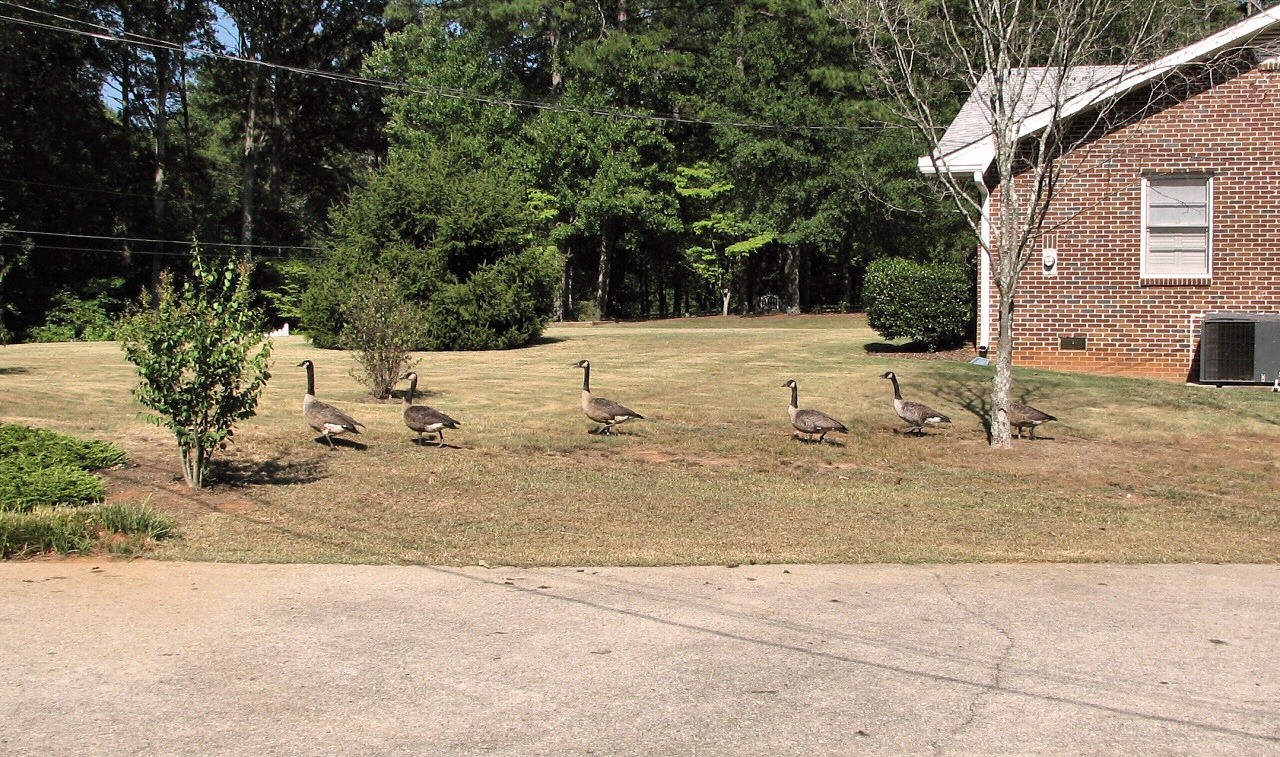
[781,380,849,444]
[297,360,366,451]
[572,360,644,436]
[399,372,461,450]
[879,371,952,437]
[1010,403,1059,440]
